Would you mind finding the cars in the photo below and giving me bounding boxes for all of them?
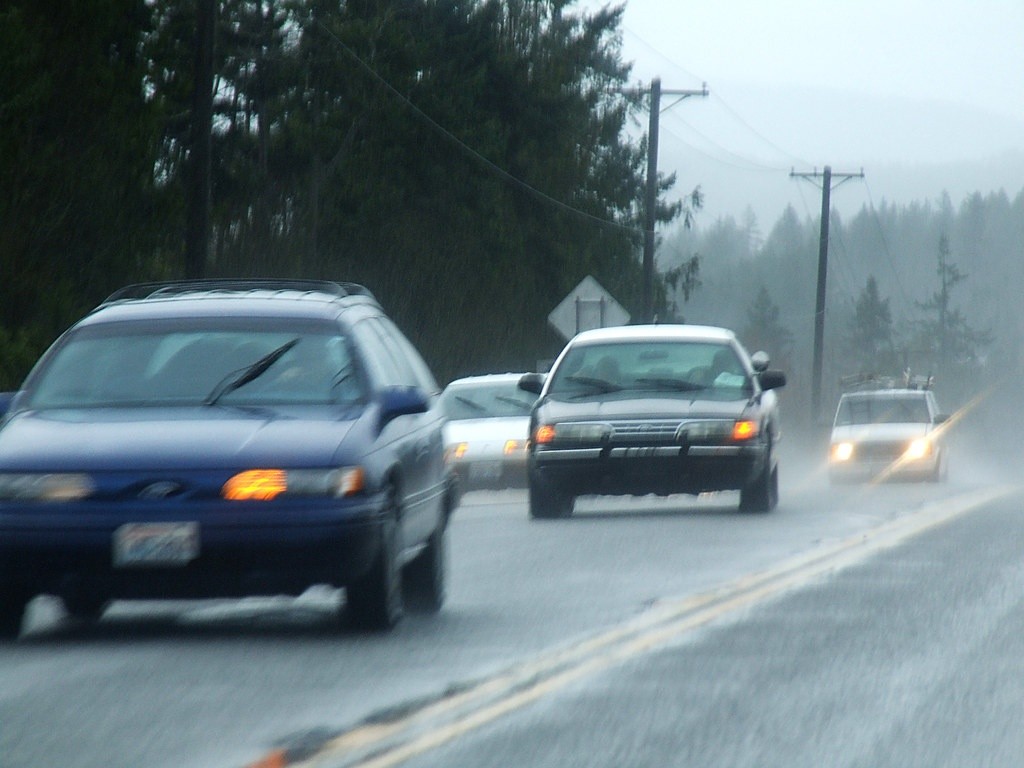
[439,371,549,489]
[816,388,952,483]
[1,274,464,644]
[515,322,788,518]
